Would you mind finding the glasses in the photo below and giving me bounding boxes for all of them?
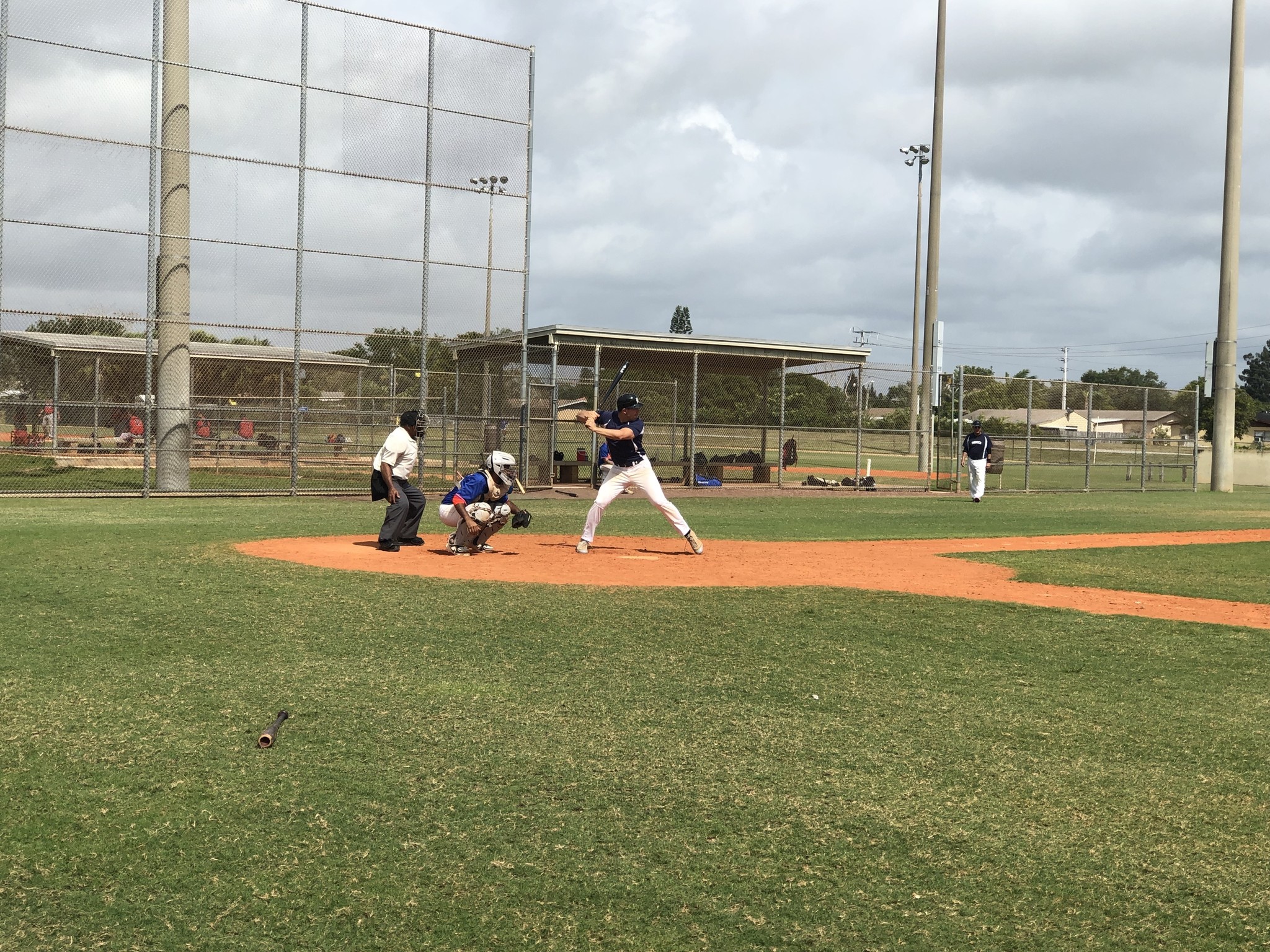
[974,426,980,429]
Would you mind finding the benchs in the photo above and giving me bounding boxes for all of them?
[57,436,291,456]
[538,460,778,485]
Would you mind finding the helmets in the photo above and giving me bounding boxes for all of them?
[485,450,517,486]
[554,451,564,461]
[972,421,982,427]
[617,394,644,409]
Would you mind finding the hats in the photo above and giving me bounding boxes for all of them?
[400,411,425,426]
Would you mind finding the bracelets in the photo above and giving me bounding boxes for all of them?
[986,458,991,463]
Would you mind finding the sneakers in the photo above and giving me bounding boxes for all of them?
[469,541,492,553]
[397,537,425,546]
[681,529,703,554]
[378,540,400,552]
[445,535,471,556]
[575,539,593,553]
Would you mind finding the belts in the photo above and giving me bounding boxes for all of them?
[614,457,643,467]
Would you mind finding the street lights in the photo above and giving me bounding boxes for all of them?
[899,143,931,456]
[469,175,509,436]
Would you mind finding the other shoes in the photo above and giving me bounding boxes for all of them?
[621,488,634,494]
[973,497,980,502]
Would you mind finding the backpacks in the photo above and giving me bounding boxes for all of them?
[782,435,798,471]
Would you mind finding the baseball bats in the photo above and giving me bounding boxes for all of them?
[480,452,487,470]
[510,468,526,495]
[457,470,464,479]
[555,490,579,498]
[585,360,630,429]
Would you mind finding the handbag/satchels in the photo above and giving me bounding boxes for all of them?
[371,469,388,501]
[802,475,840,490]
[710,454,736,463]
[735,449,763,463]
[841,477,877,492]
[680,452,708,463]
[684,473,722,488]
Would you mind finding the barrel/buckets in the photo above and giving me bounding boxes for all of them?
[985,438,1005,474]
[576,448,587,461]
[486,425,502,452]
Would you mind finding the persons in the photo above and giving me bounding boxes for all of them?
[124,410,144,455]
[576,394,703,555]
[961,420,993,503]
[230,413,254,457]
[38,392,60,440]
[192,411,211,456]
[14,394,30,447]
[371,411,426,551]
[439,451,531,556]
[111,394,130,455]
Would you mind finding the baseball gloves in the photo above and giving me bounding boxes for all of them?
[510,509,533,530]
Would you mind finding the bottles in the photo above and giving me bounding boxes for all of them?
[585,452,588,461]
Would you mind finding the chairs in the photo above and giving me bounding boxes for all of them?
[11,431,46,446]
